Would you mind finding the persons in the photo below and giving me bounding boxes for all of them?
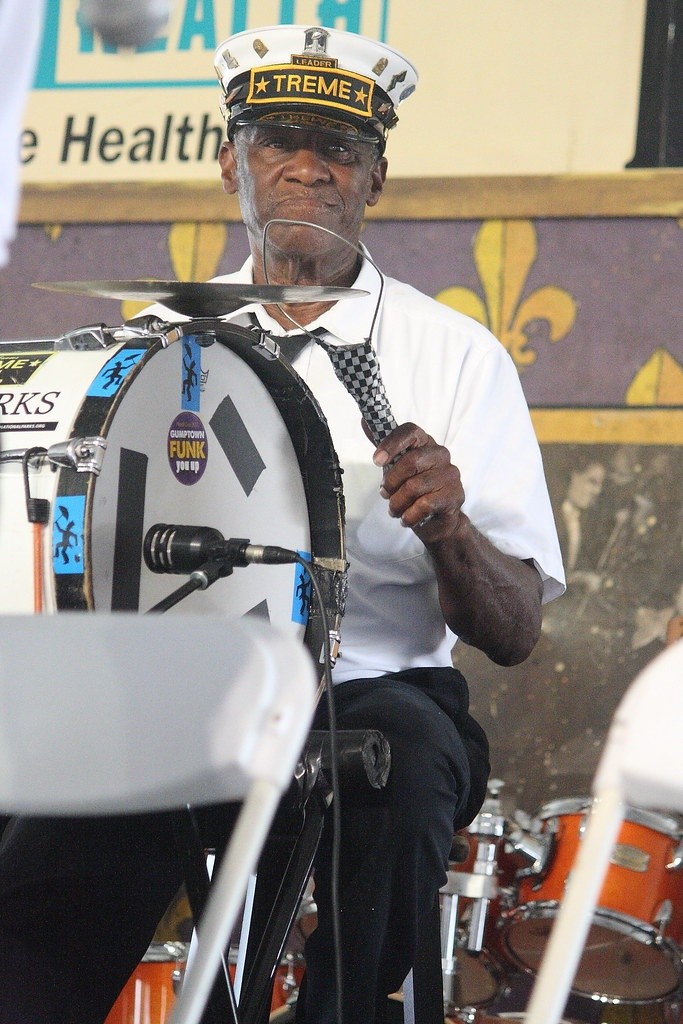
[0,23,565,1024]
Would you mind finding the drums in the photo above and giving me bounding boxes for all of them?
[298,799,530,1007]
[501,796,683,1008]
[0,320,349,695]
[99,946,308,1024]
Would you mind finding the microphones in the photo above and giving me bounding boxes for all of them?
[143,523,300,574]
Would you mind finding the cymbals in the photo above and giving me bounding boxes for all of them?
[30,278,371,321]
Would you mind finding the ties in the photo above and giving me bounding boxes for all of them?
[246,309,329,365]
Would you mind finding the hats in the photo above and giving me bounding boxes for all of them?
[211,24,419,157]
